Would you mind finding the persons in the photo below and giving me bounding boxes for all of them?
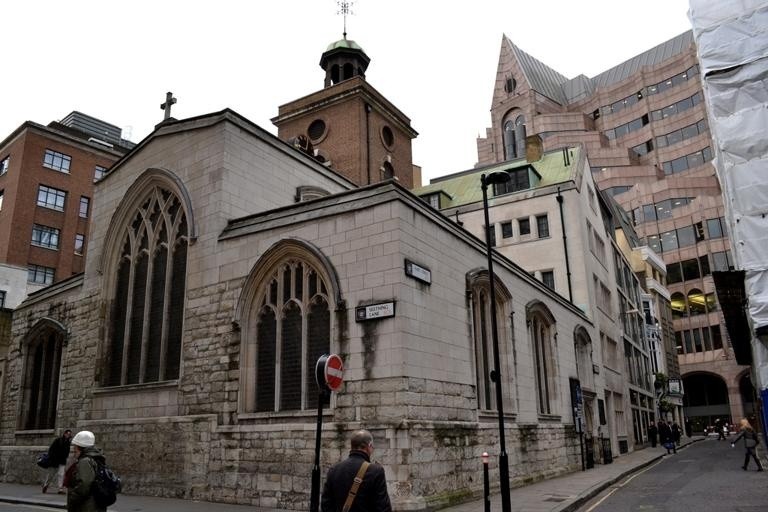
[715,418,726,440]
[318,429,393,511]
[41,429,74,495]
[648,419,681,454]
[62,429,108,512]
[730,418,764,472]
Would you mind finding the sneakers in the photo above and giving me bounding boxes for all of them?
[757,468,764,472]
[57,490,67,495]
[741,465,747,471]
[668,452,677,454]
[716,438,726,441]
[41,484,49,494]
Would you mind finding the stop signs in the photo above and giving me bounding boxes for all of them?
[324,354,345,392]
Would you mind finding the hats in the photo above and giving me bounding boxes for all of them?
[71,430,96,448]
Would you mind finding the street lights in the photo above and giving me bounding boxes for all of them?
[479,169,516,511]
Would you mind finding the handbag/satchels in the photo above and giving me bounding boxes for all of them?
[663,442,675,449]
[36,453,49,469]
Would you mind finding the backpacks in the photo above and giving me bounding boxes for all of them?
[89,457,121,507]
